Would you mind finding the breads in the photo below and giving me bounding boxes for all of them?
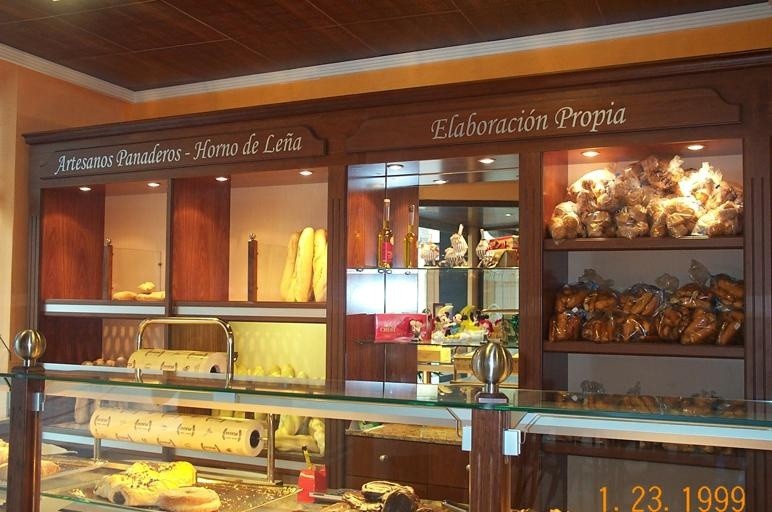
[547,156,745,240]
[0,437,61,483]
[547,260,746,344]
[280,226,328,302]
[91,460,221,512]
[321,480,434,512]
[547,390,745,420]
[113,282,166,303]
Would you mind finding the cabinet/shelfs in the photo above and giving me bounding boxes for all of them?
[343,433,471,506]
[34,163,335,471]
[530,133,757,458]
[335,148,528,445]
